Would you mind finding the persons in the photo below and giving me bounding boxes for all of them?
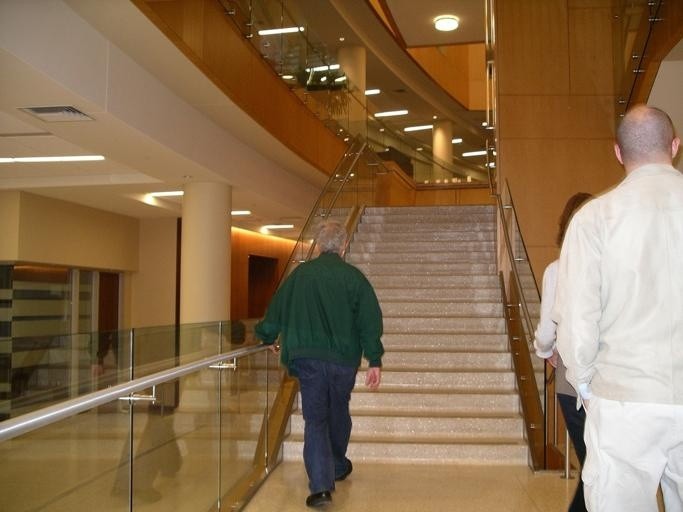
[252,218,385,509]
[550,103,682,512]
[86,319,246,504]
[532,189,598,511]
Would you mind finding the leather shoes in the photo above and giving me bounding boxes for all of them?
[306,492,332,508]
[334,457,353,482]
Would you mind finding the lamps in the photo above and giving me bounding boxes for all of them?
[432,14,460,32]
